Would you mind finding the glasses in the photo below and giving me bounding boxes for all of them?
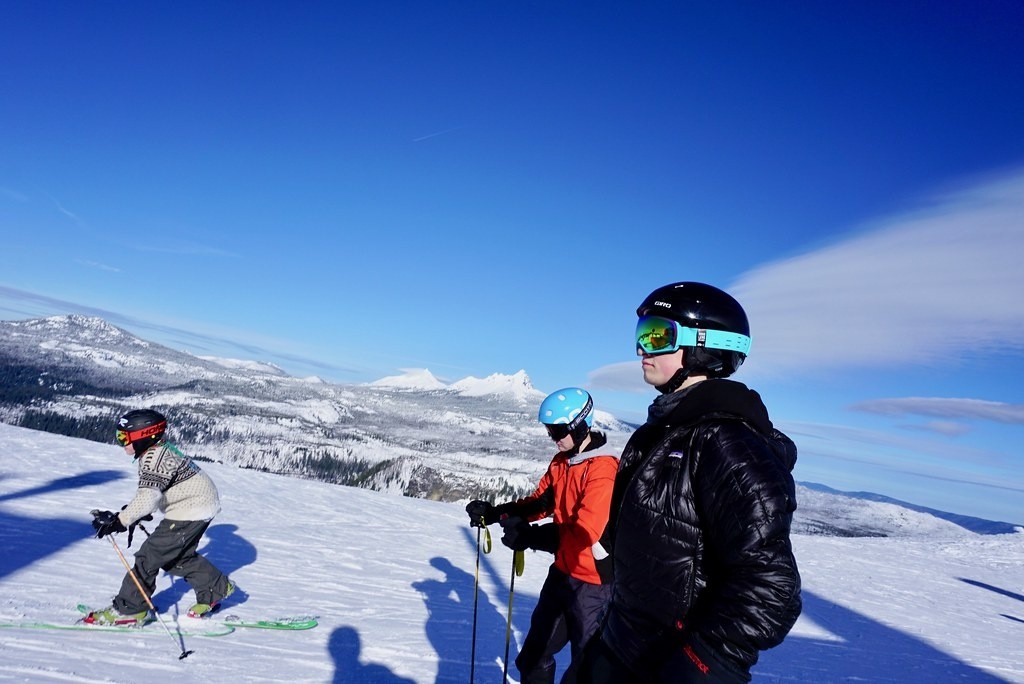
[115,429,130,446]
[634,318,681,354]
[543,422,568,442]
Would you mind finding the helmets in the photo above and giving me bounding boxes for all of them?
[638,281,751,375]
[118,408,167,458]
[540,387,593,448]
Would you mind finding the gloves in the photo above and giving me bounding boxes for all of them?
[499,517,560,555]
[467,499,517,528]
[93,510,124,537]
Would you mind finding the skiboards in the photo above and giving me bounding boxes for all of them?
[0,604,318,635]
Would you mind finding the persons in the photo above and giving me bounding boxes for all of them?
[82,409,236,626]
[465,387,623,684]
[558,282,805,684]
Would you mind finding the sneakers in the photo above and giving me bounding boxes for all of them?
[88,605,148,630]
[187,580,235,617]
